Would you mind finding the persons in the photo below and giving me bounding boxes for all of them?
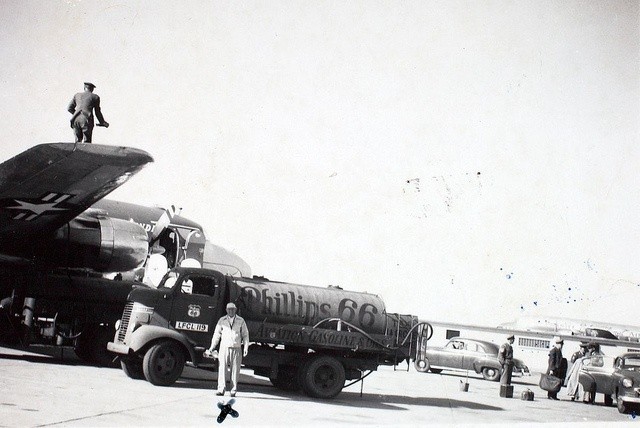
[586,342,597,355]
[546,337,564,400]
[457,342,464,350]
[570,344,588,400]
[206,302,249,397]
[68,82,109,143]
[563,352,591,404]
[498,334,515,384]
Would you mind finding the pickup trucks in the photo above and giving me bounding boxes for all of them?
[414,337,531,381]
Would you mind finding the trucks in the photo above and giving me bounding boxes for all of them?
[107,266,433,399]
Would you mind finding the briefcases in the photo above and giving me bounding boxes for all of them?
[500,384,511,398]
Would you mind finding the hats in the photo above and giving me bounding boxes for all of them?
[507,335,515,340]
[84,82,96,88]
[556,340,564,344]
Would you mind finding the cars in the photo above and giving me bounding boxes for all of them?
[579,352,640,413]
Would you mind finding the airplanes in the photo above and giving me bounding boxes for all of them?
[0,143,175,360]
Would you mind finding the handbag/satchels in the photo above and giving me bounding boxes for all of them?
[539,373,563,392]
[521,387,534,401]
